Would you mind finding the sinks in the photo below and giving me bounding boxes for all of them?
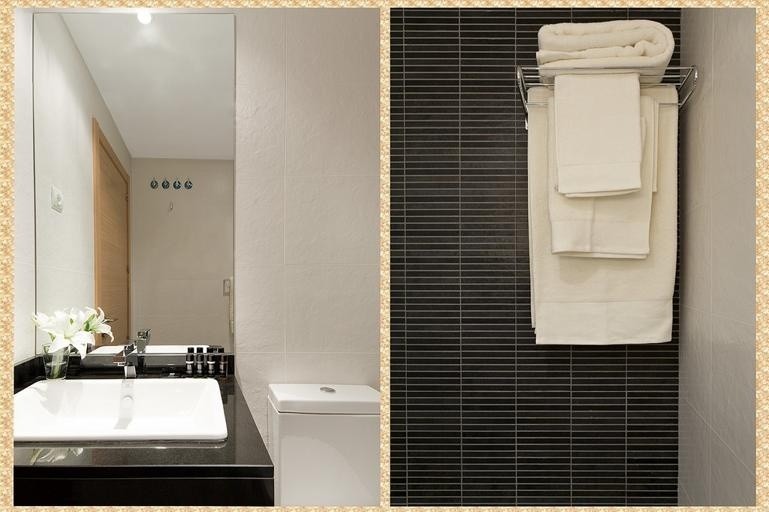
[13,380,229,444]
[87,344,209,355]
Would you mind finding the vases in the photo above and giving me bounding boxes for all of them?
[43,343,69,380]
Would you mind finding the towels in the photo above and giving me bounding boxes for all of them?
[533,20,675,85]
[521,85,680,347]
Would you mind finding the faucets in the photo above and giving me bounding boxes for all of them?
[112,341,139,379]
[133,328,152,353]
[114,380,136,433]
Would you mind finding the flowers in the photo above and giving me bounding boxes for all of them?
[32,306,96,376]
[83,306,116,343]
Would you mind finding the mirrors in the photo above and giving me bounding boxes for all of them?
[33,11,236,357]
